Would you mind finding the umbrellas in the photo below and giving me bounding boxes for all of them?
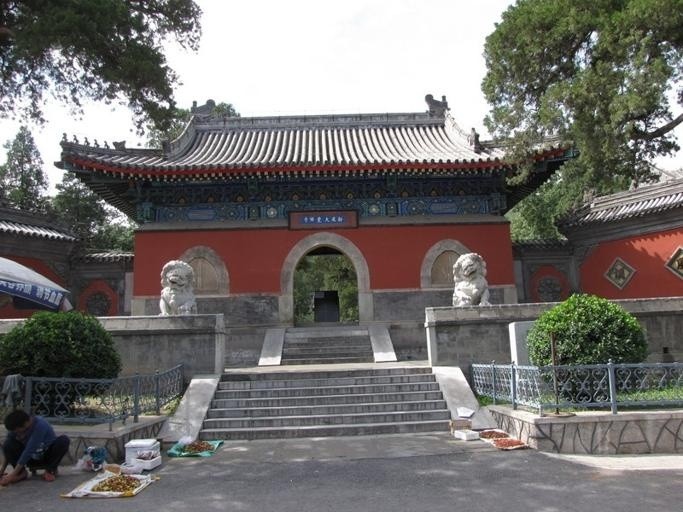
[0,257,70,310]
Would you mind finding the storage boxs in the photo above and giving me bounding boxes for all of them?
[125,439,161,465]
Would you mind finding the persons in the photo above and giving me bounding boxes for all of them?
[0,411,70,486]
